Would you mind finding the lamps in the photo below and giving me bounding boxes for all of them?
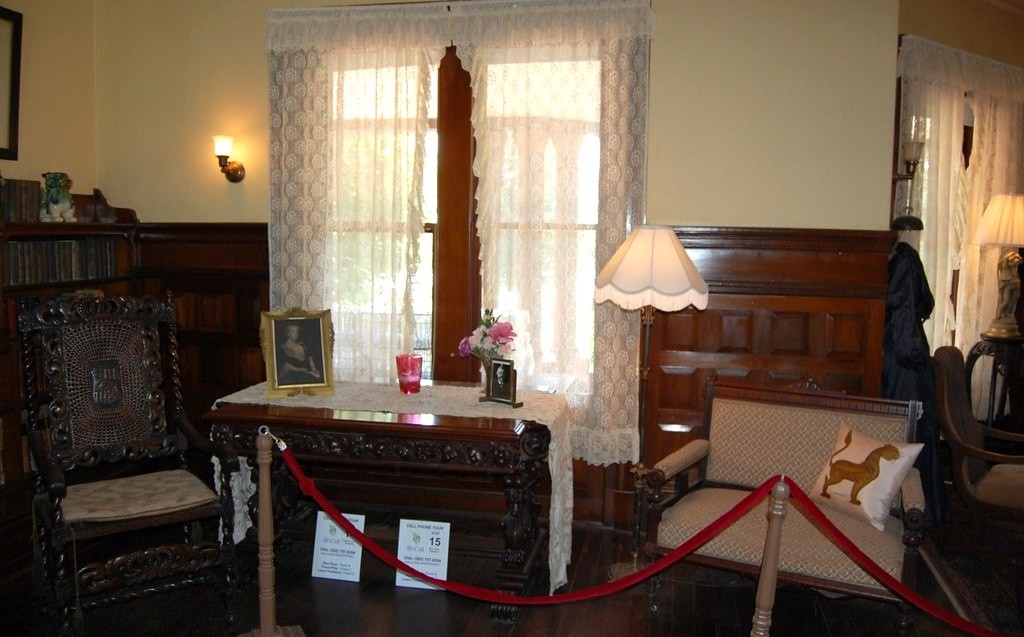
[968,192,1024,339]
[591,222,709,589]
[212,134,245,184]
[892,115,932,183]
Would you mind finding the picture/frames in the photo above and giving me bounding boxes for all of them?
[0,5,24,161]
[259,304,336,401]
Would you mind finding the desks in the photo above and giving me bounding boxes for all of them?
[963,332,1024,436]
[204,382,573,635]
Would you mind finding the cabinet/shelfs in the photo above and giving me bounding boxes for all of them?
[0,189,270,628]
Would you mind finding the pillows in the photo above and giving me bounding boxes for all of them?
[805,416,922,534]
[973,463,1024,510]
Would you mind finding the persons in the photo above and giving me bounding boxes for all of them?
[279,324,321,385]
[492,366,511,398]
[997,251,1023,319]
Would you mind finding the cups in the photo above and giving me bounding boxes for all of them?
[395,353,423,395]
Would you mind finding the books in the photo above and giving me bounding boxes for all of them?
[4,238,116,285]
[0,179,41,223]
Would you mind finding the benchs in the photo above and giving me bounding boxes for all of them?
[640,371,924,637]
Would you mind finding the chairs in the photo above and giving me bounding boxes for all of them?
[933,344,1024,568]
[19,288,240,637]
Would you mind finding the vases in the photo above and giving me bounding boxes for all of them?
[480,352,503,394]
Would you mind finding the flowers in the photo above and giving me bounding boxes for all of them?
[457,308,517,360]
